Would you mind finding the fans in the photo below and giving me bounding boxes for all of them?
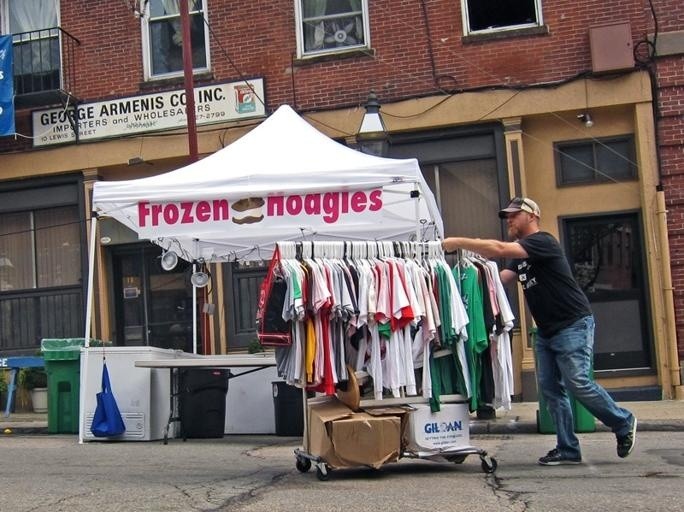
[323,20,358,48]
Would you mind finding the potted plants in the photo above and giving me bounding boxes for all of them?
[17,351,48,415]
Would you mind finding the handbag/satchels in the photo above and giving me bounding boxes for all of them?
[255,244,292,347]
[90,363,125,436]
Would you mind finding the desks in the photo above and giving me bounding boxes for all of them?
[134,357,277,443]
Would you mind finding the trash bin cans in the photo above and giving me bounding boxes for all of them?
[41,338,113,434]
[528,328,595,433]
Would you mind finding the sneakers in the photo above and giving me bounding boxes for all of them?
[538,448,581,465]
[617,412,636,458]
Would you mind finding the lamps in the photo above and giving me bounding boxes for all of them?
[581,81,594,128]
[354,88,391,142]
[159,243,211,287]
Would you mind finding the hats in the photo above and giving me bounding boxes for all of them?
[498,197,540,220]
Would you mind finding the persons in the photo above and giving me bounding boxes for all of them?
[441,196,638,467]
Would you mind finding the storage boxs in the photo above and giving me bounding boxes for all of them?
[306,394,472,468]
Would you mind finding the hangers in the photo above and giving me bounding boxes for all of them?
[278,240,499,278]
